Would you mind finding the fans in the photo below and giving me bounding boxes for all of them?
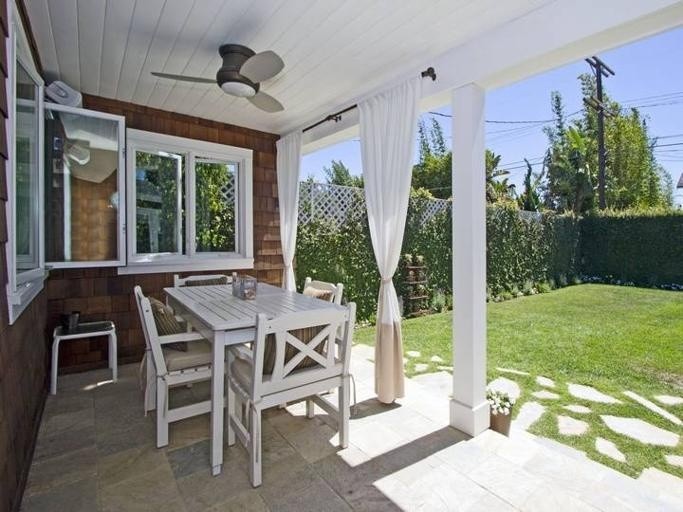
[150,44,285,113]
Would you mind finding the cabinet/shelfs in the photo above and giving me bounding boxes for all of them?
[403,266,429,318]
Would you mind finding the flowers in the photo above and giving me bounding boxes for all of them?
[486,388,516,416]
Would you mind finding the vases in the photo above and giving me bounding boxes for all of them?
[489,404,512,436]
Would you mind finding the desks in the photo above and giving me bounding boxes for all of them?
[49,320,118,395]
[161,281,350,478]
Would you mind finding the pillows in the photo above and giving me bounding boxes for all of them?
[145,290,190,352]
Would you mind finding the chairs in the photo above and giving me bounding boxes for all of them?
[132,282,226,449]
[275,274,344,420]
[224,302,356,488]
[172,269,237,288]
